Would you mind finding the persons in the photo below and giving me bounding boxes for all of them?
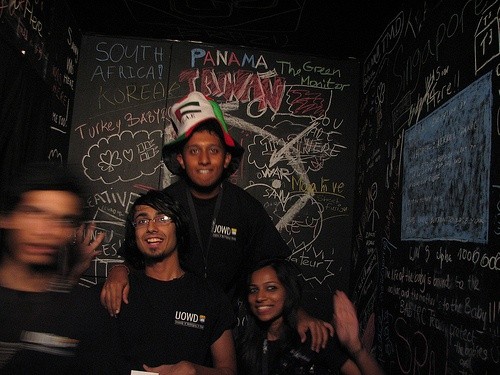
[232,256,383,375]
[100,90,334,352]
[94,189,236,375]
[0,181,128,375]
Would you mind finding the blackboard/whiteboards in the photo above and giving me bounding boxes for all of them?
[68,35,361,304]
[0,0,76,188]
[361,1,499,375]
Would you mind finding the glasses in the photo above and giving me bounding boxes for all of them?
[131,212,174,229]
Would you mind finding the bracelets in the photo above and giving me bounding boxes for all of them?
[353,345,366,357]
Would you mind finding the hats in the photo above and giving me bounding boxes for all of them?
[162,90,245,181]
